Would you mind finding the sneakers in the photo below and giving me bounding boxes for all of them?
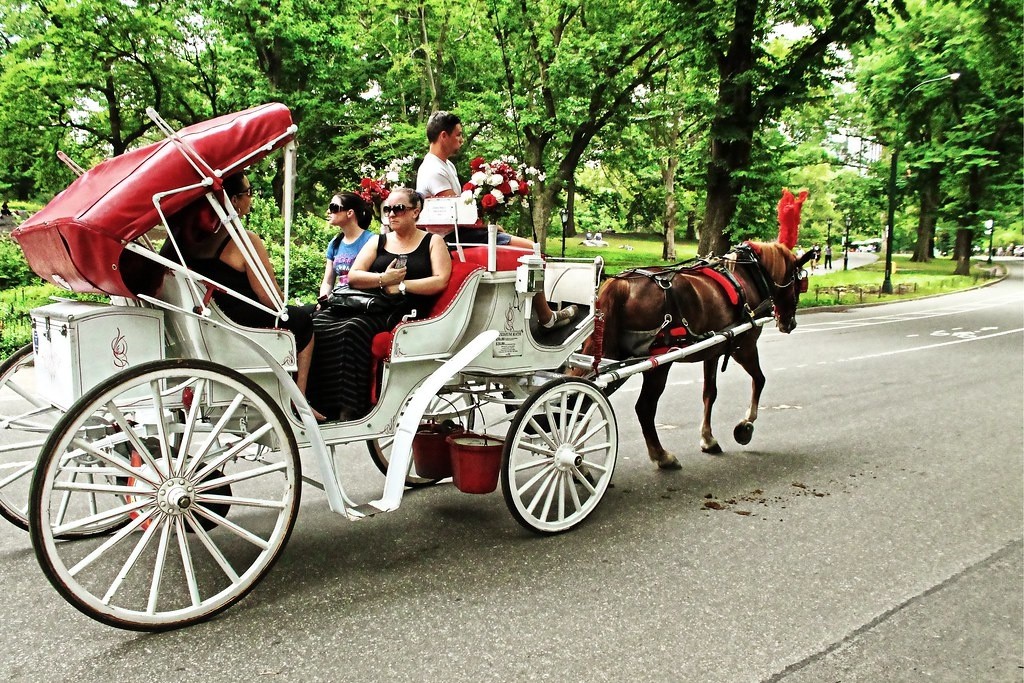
[538,305,579,332]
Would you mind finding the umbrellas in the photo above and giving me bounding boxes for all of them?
[851,238,881,245]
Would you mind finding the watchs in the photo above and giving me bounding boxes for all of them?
[399,282,406,295]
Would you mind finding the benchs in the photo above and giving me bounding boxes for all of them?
[109,265,300,374]
[370,258,487,434]
[415,194,547,284]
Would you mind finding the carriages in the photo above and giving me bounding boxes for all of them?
[0,100,816,634]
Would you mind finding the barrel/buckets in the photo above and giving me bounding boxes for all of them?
[445,405,504,493]
[412,394,464,479]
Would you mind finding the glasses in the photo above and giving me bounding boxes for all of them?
[383,204,416,216]
[329,203,349,213]
[234,186,253,198]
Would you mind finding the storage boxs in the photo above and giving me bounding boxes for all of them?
[29,301,165,412]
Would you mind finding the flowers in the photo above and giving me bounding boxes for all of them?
[352,153,420,205]
[461,155,547,224]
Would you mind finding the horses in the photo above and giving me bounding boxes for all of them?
[568,241,816,483]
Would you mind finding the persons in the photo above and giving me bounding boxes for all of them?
[594,231,602,240]
[417,112,578,330]
[991,242,1015,256]
[859,244,878,252]
[810,243,821,275]
[316,191,375,310]
[312,188,451,421]
[161,173,327,424]
[586,231,593,239]
[797,245,805,255]
[824,244,832,269]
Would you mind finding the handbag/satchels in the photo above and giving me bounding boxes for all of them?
[328,283,403,318]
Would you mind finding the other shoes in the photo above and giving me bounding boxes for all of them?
[292,399,326,424]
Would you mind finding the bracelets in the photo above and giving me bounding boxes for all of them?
[379,274,383,289]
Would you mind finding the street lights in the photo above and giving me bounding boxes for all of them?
[843,217,853,271]
[558,208,570,262]
[826,218,833,247]
